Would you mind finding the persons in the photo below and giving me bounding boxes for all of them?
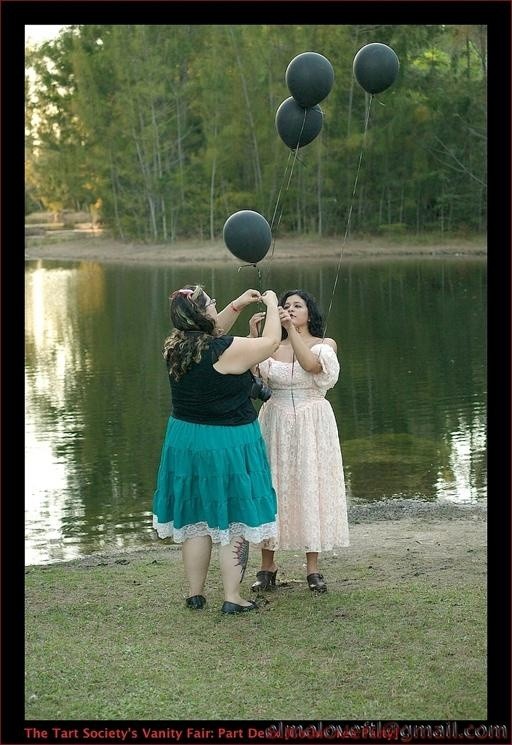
[151,283,284,615]
[245,287,352,592]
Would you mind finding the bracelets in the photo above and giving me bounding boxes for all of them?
[229,301,241,314]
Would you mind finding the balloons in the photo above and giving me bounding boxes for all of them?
[353,41,400,94]
[285,51,334,108]
[223,208,273,267]
[275,95,323,152]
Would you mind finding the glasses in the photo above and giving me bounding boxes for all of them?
[205,298,216,308]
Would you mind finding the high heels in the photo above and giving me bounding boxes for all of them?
[251,568,279,591]
[307,573,327,592]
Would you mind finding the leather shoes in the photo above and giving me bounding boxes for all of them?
[186,595,206,609]
[221,600,255,614]
